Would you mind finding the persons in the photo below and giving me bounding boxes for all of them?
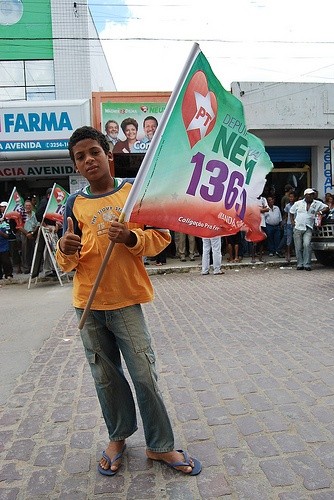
[130,116,158,153]
[112,118,139,153]
[54,126,201,476]
[0,188,63,279]
[104,120,123,154]
[144,188,334,275]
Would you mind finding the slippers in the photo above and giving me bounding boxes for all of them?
[97,443,127,475]
[149,450,202,476]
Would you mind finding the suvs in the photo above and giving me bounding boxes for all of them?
[310,207,334,268]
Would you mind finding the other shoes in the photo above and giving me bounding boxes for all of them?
[180,257,186,262]
[214,272,225,275]
[190,256,195,261]
[24,270,30,274]
[234,258,241,262]
[32,274,38,278]
[276,251,285,258]
[297,267,304,270]
[251,260,255,263]
[227,258,234,262]
[259,258,265,262]
[305,267,311,271]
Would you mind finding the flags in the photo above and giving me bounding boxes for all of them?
[44,184,71,225]
[124,48,274,241]
[0,189,36,229]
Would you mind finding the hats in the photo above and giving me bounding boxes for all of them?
[303,188,315,195]
[0,202,8,206]
